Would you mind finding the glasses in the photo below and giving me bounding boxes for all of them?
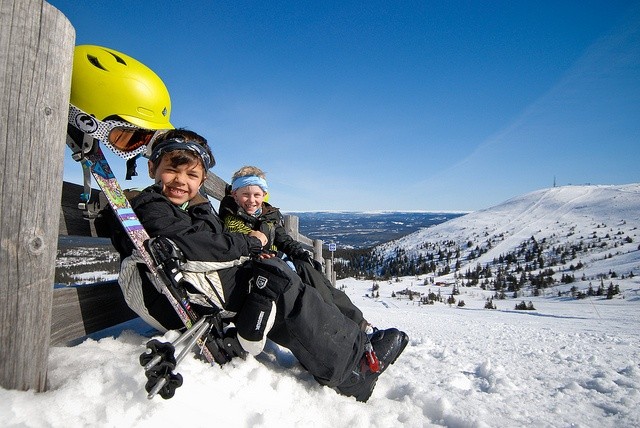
[68,105,165,161]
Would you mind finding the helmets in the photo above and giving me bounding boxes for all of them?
[68,44,175,131]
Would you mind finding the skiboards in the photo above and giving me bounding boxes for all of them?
[85,146,231,365]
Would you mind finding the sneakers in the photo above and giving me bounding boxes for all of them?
[337,328,402,404]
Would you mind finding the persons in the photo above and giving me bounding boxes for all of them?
[218,164,380,340]
[109,130,408,404]
